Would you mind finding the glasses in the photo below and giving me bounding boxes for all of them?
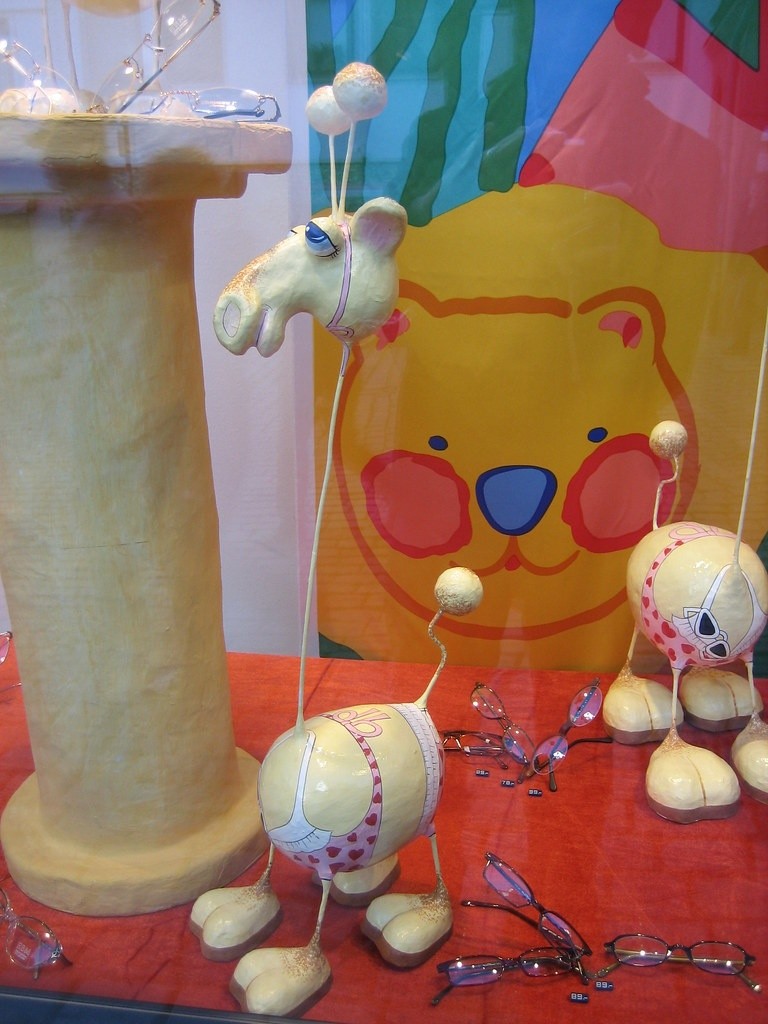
[439,730,511,768]
[597,933,763,993]
[0,890,73,980]
[527,676,613,794]
[0,631,21,691]
[99,87,281,123]
[430,947,591,1004]
[86,0,221,113]
[0,40,78,116]
[470,682,540,784]
[462,849,591,963]
